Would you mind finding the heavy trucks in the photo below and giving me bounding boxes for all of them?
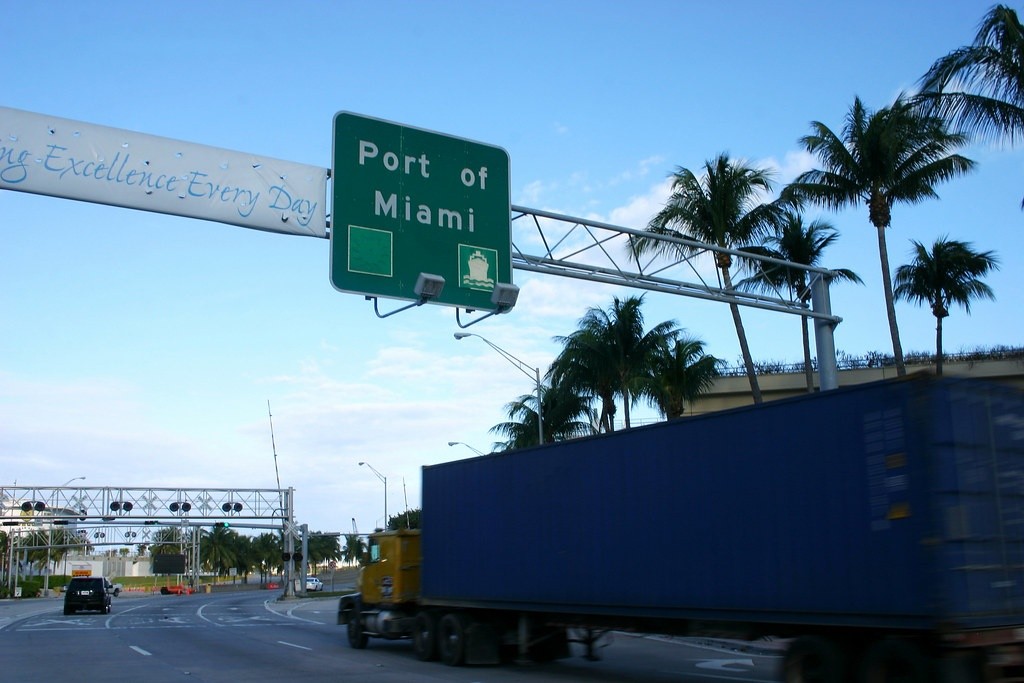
[338,373,1024,672]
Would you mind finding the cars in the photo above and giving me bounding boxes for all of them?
[305,578,324,591]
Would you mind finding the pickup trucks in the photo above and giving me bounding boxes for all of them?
[108,580,124,597]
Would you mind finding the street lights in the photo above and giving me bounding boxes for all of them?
[448,441,483,454]
[452,329,545,449]
[36,477,86,517]
[359,462,388,531]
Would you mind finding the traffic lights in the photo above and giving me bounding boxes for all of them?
[145,521,158,525]
[214,522,229,528]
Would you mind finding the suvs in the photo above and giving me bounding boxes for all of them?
[65,574,112,616]
